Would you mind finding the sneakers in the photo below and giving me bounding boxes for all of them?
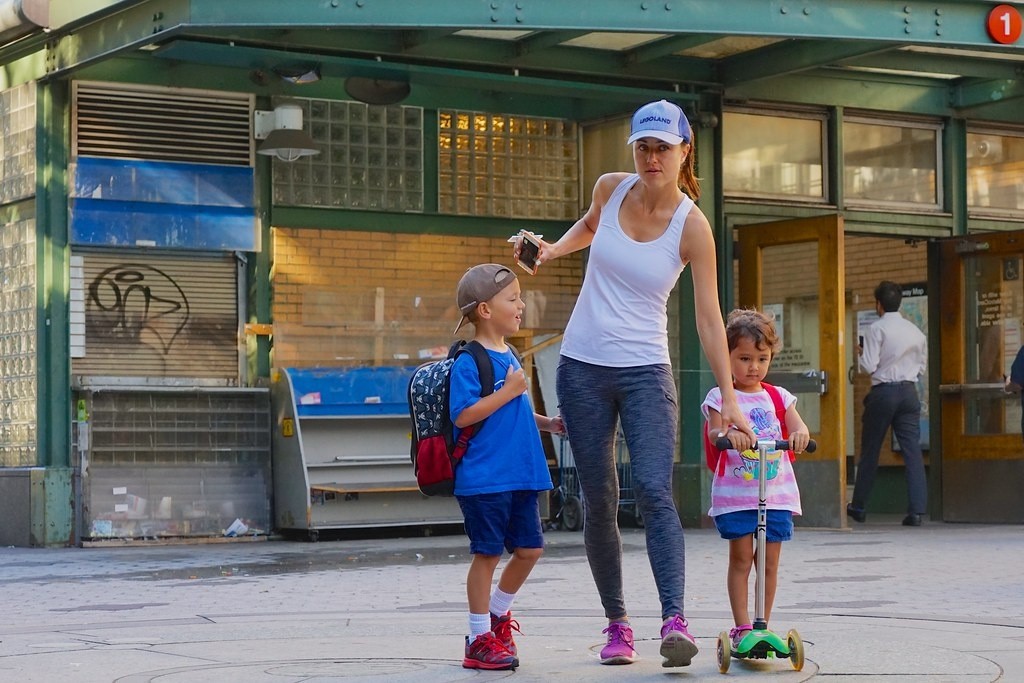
[462,631,519,668]
[660,614,699,667]
[489,610,525,667]
[600,621,640,664]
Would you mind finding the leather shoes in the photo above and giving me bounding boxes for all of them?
[847,503,865,522]
[903,514,921,525]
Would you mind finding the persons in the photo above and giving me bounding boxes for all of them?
[514,100,756,667]
[1005,346,1024,439]
[449,263,570,670]
[700,310,810,652]
[846,281,927,526]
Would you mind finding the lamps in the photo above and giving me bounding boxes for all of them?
[251,101,322,163]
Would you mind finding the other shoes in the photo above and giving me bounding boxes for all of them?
[729,625,754,647]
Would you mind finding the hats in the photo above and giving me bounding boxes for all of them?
[626,99,691,145]
[453,264,518,334]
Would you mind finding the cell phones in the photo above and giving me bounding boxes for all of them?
[517,232,543,275]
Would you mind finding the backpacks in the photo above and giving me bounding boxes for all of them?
[704,382,797,477]
[407,339,496,496]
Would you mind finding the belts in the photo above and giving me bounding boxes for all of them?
[880,381,913,385]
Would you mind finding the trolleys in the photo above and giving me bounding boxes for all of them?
[540,393,645,532]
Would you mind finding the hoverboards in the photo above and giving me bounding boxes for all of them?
[714,437,818,675]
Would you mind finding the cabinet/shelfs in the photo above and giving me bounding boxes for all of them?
[272,365,553,541]
